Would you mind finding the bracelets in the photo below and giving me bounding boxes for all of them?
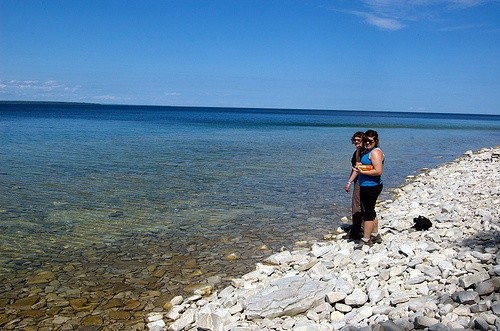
[347,182,351,185]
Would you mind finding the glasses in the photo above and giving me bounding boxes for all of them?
[363,139,374,143]
[353,139,362,142]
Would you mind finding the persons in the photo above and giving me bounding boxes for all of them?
[342,131,364,239]
[352,130,386,250]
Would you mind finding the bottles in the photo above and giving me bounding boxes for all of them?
[355,165,373,171]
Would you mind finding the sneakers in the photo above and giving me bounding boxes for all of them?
[370,234,381,244]
[344,225,353,232]
[354,239,373,250]
[342,229,361,239]
